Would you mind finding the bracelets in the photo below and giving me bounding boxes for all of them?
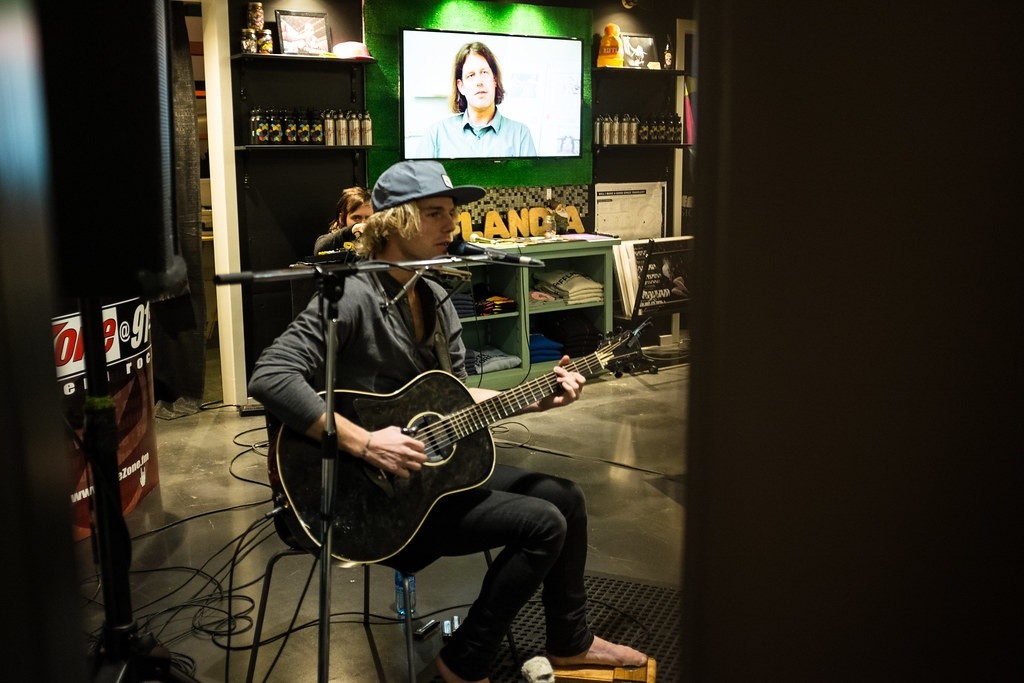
[361,432,374,458]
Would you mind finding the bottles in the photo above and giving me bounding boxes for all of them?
[240,28,257,53]
[247,2,264,29]
[544,217,556,237]
[249,105,373,145]
[663,42,672,69]
[257,29,274,54]
[394,569,416,615]
[593,113,681,144]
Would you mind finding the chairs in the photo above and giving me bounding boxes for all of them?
[246,408,517,683]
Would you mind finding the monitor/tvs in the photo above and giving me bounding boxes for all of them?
[396,25,585,160]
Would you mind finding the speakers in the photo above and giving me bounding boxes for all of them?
[31,0,184,291]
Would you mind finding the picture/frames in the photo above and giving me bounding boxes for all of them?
[619,31,659,69]
[274,9,333,55]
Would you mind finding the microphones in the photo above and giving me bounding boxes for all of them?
[446,241,547,267]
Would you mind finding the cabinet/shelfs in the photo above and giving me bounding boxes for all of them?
[442,234,621,390]
[231,52,379,187]
[593,65,694,172]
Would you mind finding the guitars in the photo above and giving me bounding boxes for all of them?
[267,317,665,565]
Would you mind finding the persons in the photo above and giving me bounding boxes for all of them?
[415,41,537,157]
[314,186,374,257]
[248,160,649,683]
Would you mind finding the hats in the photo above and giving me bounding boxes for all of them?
[371,160,487,212]
